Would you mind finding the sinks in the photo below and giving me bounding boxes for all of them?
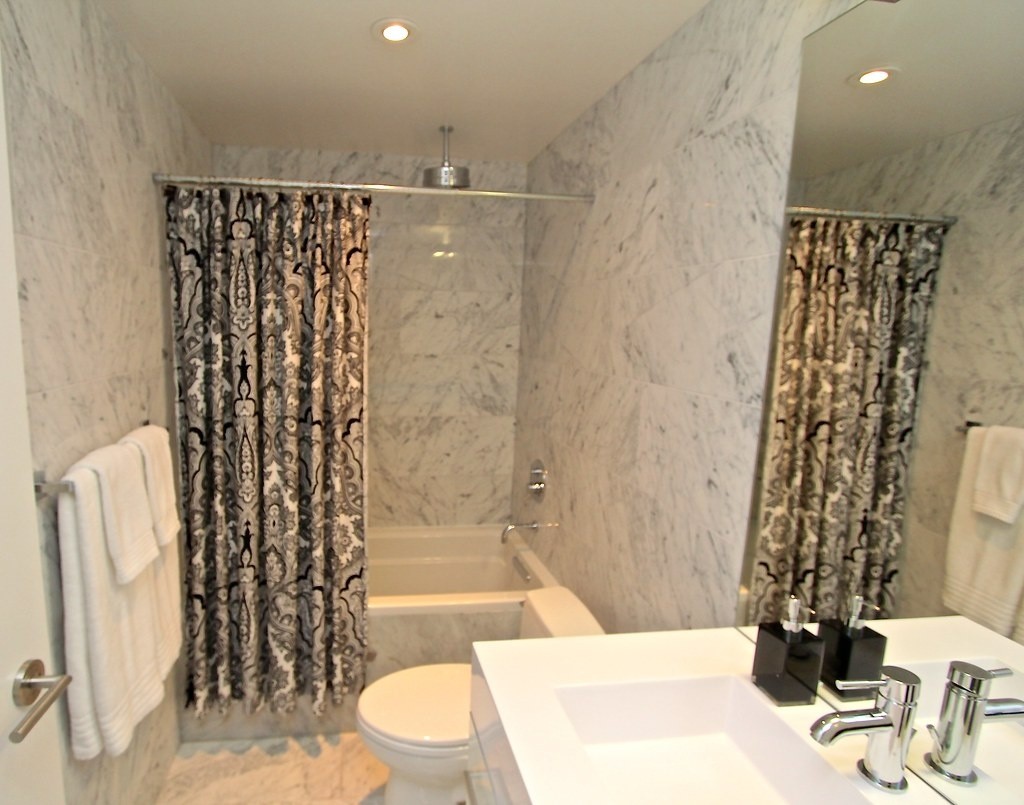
[542,670,890,805]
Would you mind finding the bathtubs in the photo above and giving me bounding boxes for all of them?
[176,522,564,745]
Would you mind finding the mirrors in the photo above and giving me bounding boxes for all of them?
[732,2,1024,805]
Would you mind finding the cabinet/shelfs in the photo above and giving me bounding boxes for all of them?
[463,712,499,805]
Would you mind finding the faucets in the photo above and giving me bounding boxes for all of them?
[499,519,542,546]
[808,666,924,796]
[928,659,1023,793]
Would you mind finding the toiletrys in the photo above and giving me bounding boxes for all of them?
[749,593,827,703]
[813,595,890,705]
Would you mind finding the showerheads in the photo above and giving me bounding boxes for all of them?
[421,165,477,192]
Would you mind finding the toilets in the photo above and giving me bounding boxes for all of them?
[351,581,609,805]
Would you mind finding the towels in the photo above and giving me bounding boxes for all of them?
[968,420,1023,526]
[122,424,187,548]
[55,466,166,764]
[939,421,1024,640]
[64,444,165,585]
[117,431,185,684]
